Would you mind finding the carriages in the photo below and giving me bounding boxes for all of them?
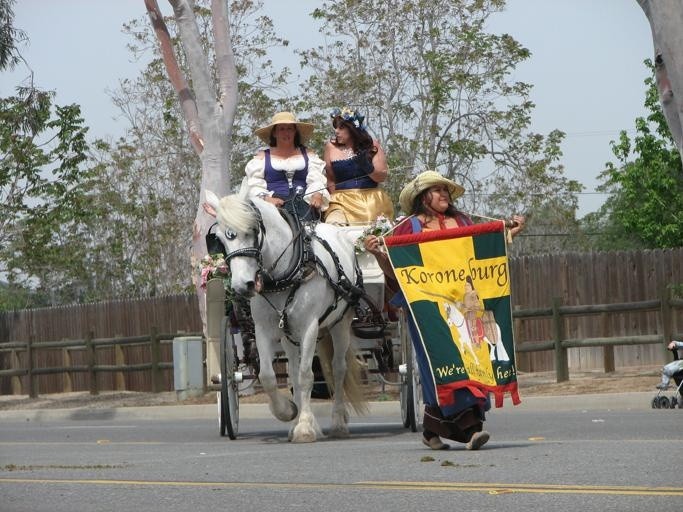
[198,181,423,437]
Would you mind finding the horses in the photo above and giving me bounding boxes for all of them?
[443,301,509,366]
[204,175,373,444]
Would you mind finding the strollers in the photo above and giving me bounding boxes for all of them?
[652,340,682,410]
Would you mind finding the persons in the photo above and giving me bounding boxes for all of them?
[454,275,480,348]
[655,340,682,392]
[362,169,525,454]
[322,105,395,226]
[242,110,329,222]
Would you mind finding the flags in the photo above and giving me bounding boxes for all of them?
[380,220,522,409]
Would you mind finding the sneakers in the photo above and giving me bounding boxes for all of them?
[656,384,669,390]
[467,431,489,450]
[423,435,450,450]
[386,289,400,321]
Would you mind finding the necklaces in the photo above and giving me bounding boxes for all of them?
[342,147,353,158]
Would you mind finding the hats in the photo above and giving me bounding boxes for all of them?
[399,170,465,214]
[254,112,314,145]
